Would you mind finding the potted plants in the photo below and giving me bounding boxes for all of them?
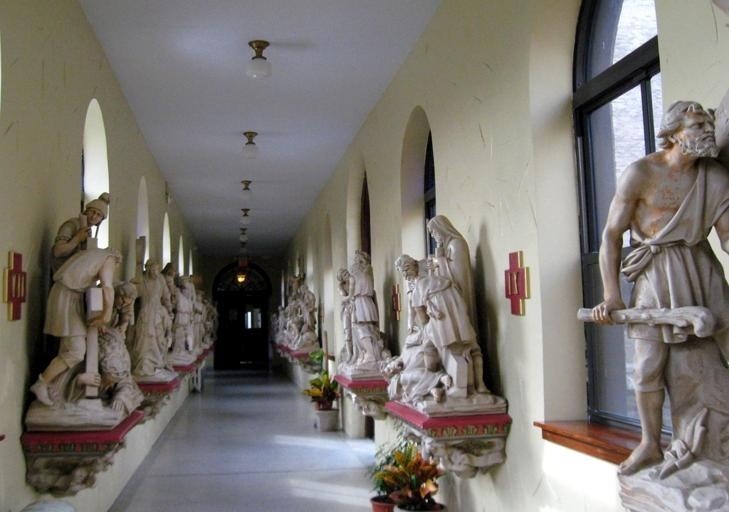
[304,370,341,432]
[368,435,444,512]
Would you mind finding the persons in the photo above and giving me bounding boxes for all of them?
[50,190,113,265]
[711,89,729,146]
[29,246,122,407]
[163,262,219,358]
[379,334,452,403]
[135,257,171,377]
[394,253,434,332]
[426,214,479,326]
[265,286,321,350]
[335,247,375,366]
[412,275,490,393]
[114,280,135,332]
[590,97,729,478]
[66,333,144,413]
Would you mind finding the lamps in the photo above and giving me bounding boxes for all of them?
[239,180,252,243]
[242,131,258,146]
[249,40,271,76]
